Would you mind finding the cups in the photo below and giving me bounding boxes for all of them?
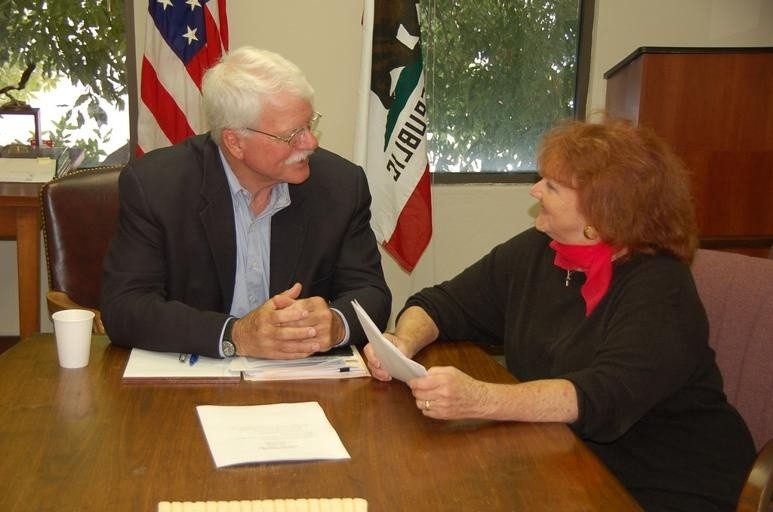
[50,308,97,369]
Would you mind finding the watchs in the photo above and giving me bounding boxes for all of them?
[221,317,238,357]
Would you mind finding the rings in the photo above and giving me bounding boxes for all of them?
[425,401,430,411]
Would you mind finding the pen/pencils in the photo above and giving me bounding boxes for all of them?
[179,354,187,362]
[190,354,198,364]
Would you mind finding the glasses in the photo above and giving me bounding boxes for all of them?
[243,111,321,149]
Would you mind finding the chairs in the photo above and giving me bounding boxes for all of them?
[689,247,772,511]
[38,162,156,335]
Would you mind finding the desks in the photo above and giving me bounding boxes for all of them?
[0,155,57,343]
[0,332,645,512]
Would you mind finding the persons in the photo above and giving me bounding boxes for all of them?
[363,116,756,512]
[99,43,394,360]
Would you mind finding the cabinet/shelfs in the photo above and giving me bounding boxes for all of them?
[602,45,772,248]
[0,100,84,178]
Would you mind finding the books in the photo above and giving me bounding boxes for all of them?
[120,344,374,384]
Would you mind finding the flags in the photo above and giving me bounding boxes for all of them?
[360,1,434,275]
[136,1,231,158]
